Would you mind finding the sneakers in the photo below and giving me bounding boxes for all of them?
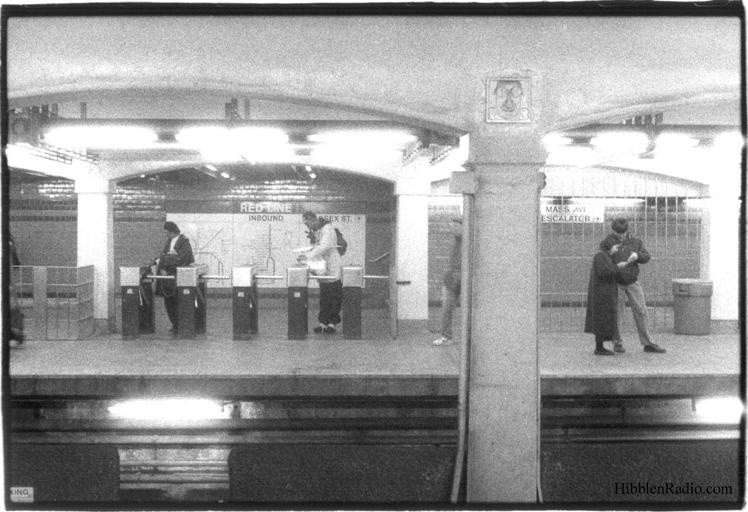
[645,345,665,353]
[315,321,337,332]
[614,345,625,352]
[433,337,453,346]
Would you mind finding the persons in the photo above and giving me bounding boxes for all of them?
[605,218,669,353]
[430,215,462,347]
[143,222,196,335]
[582,233,628,356]
[293,209,345,334]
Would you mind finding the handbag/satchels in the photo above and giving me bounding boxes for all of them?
[336,229,347,255]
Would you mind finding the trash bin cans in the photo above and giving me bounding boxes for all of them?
[671,278,713,335]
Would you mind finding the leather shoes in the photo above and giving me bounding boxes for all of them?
[595,348,614,355]
